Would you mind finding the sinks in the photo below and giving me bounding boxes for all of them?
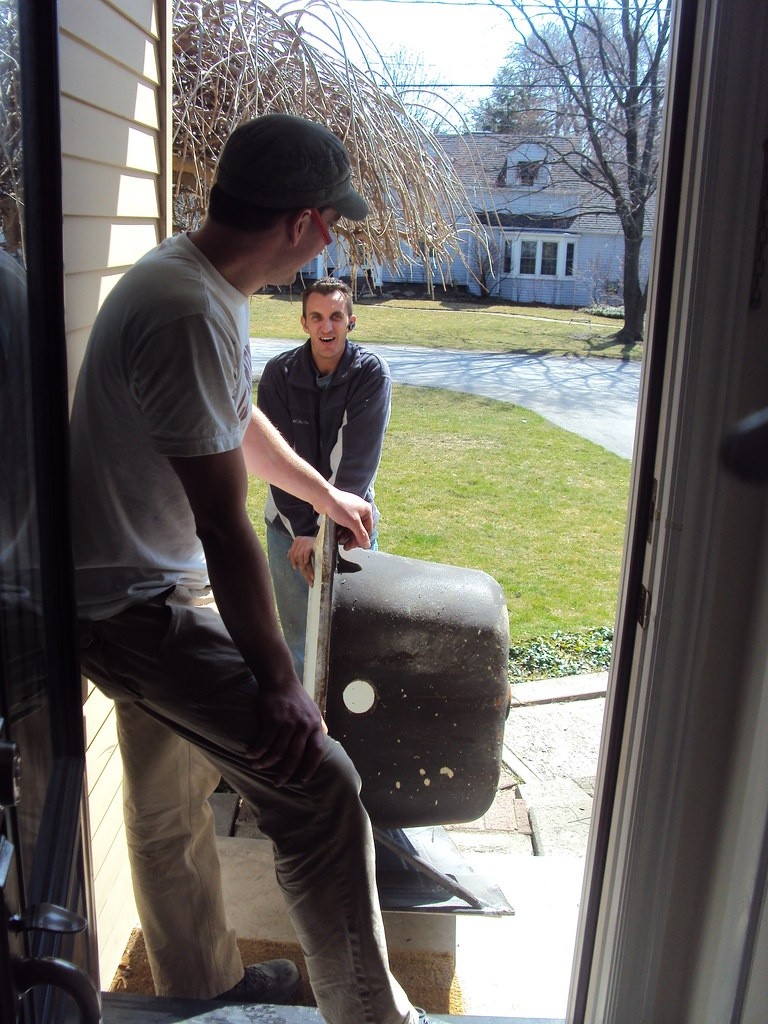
[300,509,517,832]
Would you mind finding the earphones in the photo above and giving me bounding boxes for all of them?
[349,323,355,330]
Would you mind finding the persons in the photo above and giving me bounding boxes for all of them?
[1,247,70,1024]
[257,276,390,684]
[72,113,449,1024]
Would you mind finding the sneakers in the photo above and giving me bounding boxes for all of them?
[214,957,302,1009]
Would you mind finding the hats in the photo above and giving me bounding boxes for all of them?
[207,114,369,221]
